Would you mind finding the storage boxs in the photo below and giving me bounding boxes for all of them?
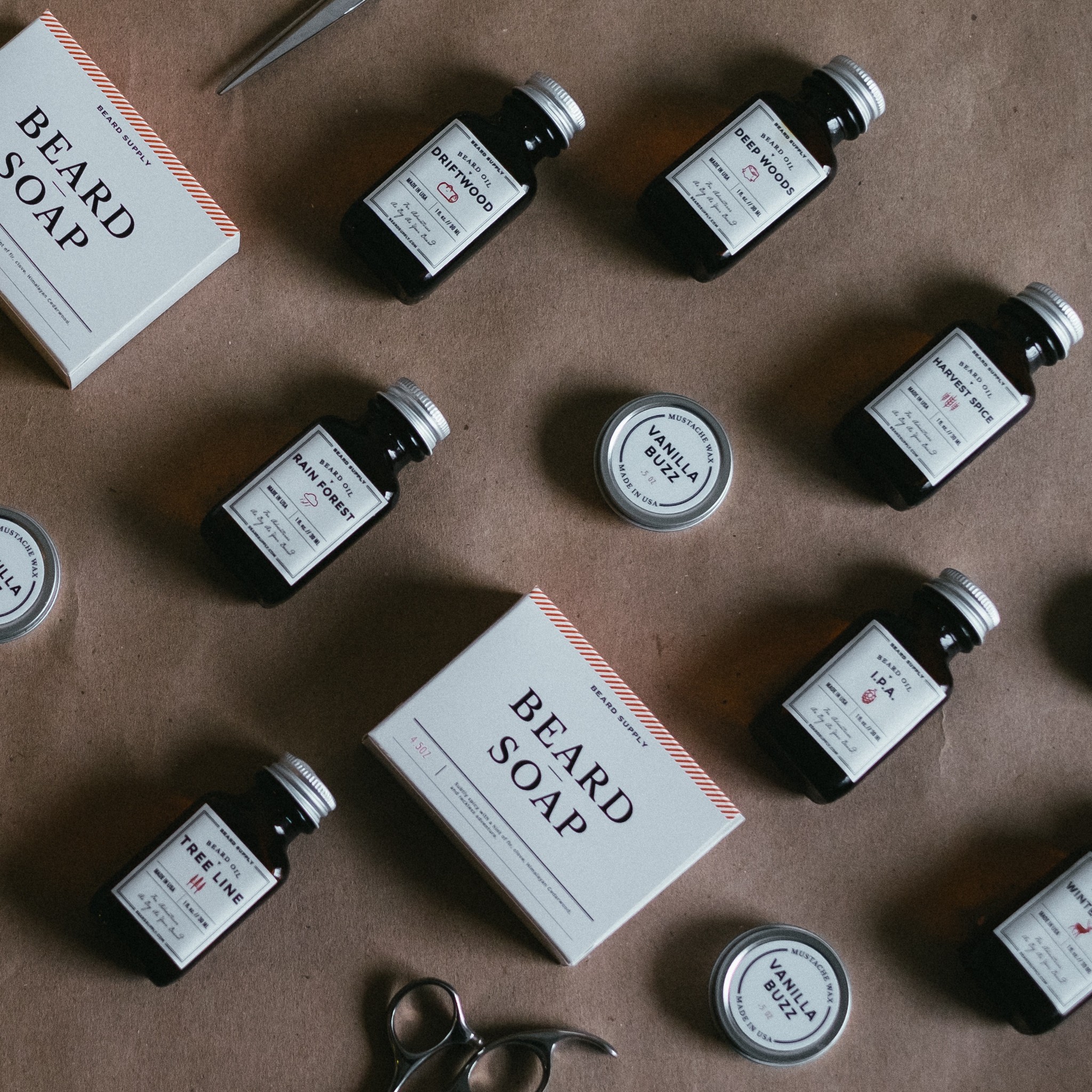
[360,586,744,971]
[0,7,241,390]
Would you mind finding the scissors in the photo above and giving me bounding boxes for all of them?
[214,0,367,96]
[380,977,619,1092]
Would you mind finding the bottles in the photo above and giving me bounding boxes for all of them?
[113,754,339,982]
[985,852,1092,1038]
[847,278,1092,508]
[638,50,896,285]
[209,374,456,613]
[764,564,1009,807]
[344,65,591,306]
